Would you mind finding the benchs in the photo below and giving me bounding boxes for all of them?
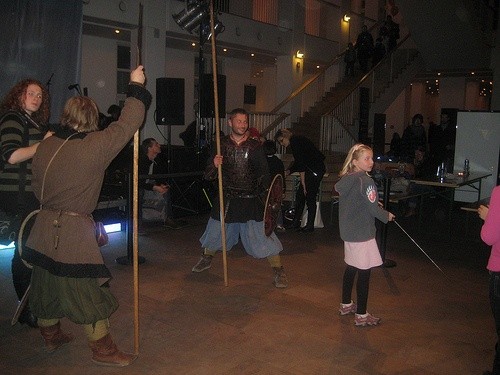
[460,196,491,232]
[388,190,426,227]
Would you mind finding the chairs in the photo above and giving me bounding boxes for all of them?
[165,149,202,215]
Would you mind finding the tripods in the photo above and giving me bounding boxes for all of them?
[162,118,214,215]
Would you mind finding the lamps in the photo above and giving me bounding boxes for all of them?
[171,0,223,44]
[344,11,351,22]
[296,49,304,58]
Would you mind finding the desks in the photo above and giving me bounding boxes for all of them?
[409,172,493,233]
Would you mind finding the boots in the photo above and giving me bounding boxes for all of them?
[90,333,138,366]
[297,205,317,232]
[41,320,73,353]
[285,201,304,229]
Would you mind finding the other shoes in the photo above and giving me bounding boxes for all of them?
[162,217,180,229]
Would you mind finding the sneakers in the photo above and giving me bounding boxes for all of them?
[272,266,288,287]
[192,252,214,272]
[338,300,358,316]
[355,313,381,326]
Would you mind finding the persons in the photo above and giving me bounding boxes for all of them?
[248,126,286,231]
[344,15,400,80]
[20,65,152,367]
[274,128,325,232]
[139,137,184,229]
[429,110,453,157]
[399,114,427,159]
[334,143,395,327]
[399,145,427,217]
[0,77,56,329]
[388,132,400,159]
[478,183,500,375]
[191,107,288,288]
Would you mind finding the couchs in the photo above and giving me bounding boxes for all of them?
[101,146,169,224]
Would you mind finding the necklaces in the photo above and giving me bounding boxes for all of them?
[230,131,246,143]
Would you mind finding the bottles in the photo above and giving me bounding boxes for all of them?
[436,161,445,181]
[464,159,470,175]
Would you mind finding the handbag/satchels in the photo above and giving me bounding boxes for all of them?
[0,193,24,246]
[300,201,324,228]
[95,222,109,246]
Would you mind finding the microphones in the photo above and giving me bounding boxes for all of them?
[68,84,78,90]
[46,73,54,85]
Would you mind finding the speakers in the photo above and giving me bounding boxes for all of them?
[201,74,226,117]
[156,78,184,125]
